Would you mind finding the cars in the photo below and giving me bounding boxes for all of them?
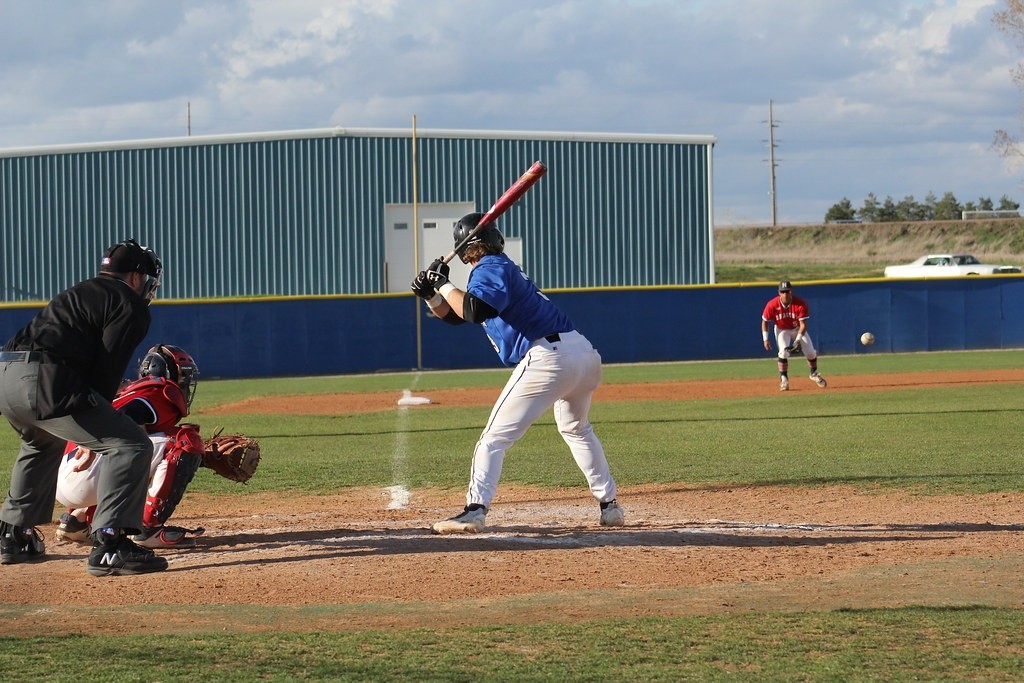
[883,252,1021,277]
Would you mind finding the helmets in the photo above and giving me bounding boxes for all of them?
[140,343,200,406]
[778,281,792,291]
[99,238,163,309]
[453,213,505,264]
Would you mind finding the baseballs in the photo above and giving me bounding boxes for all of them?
[861,333,875,347]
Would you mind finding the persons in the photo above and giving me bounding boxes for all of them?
[55,344,263,549]
[762,280,827,392]
[0,238,168,576]
[413,213,625,531]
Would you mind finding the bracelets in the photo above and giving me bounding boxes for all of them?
[437,282,456,299]
[796,333,802,341]
[763,331,769,341]
[424,293,442,309]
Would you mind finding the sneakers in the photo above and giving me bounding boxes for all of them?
[55,513,96,543]
[87,538,169,578]
[132,524,185,547]
[599,501,625,525]
[430,504,488,534]
[780,381,789,391]
[0,522,45,564]
[809,373,827,388]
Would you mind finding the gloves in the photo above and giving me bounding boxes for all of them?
[426,255,451,290]
[411,271,436,300]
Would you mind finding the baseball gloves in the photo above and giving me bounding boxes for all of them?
[784,340,802,354]
[202,434,261,483]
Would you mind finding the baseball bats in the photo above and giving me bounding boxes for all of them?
[443,159,546,261]
